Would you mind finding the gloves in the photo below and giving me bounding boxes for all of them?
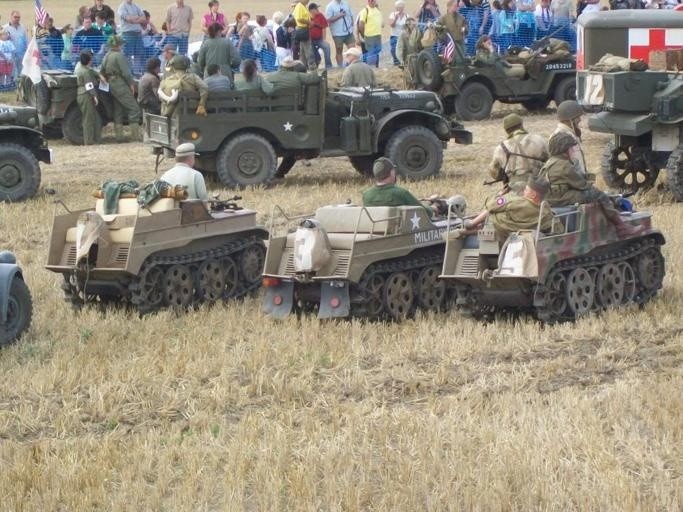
[196,106,207,116]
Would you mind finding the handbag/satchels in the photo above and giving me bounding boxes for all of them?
[295,30,308,41]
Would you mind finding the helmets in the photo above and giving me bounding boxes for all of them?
[108,35,125,46]
[167,56,190,69]
[549,131,578,155]
[504,113,523,130]
[557,101,584,121]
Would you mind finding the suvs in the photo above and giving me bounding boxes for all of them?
[575,9,682,199]
[398,40,578,119]
[0,103,54,203]
[0,244,34,350]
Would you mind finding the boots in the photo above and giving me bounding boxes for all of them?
[114,124,130,143]
[612,213,644,239]
[129,122,143,141]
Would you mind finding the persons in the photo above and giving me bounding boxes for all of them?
[442,177,565,239]
[362,157,434,220]
[488,112,548,196]
[535,132,645,238]
[0,0,682,147]
[158,142,208,200]
[548,101,586,174]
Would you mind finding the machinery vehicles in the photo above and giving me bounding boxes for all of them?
[255,195,469,320]
[44,182,269,307]
[446,191,665,315]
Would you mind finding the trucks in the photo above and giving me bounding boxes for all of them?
[142,68,472,190]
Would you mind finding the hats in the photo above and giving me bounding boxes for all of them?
[175,143,200,156]
[281,57,294,67]
[344,48,360,57]
[528,175,548,193]
[309,3,320,9]
[373,157,397,180]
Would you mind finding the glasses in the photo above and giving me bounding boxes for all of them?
[13,16,20,18]
